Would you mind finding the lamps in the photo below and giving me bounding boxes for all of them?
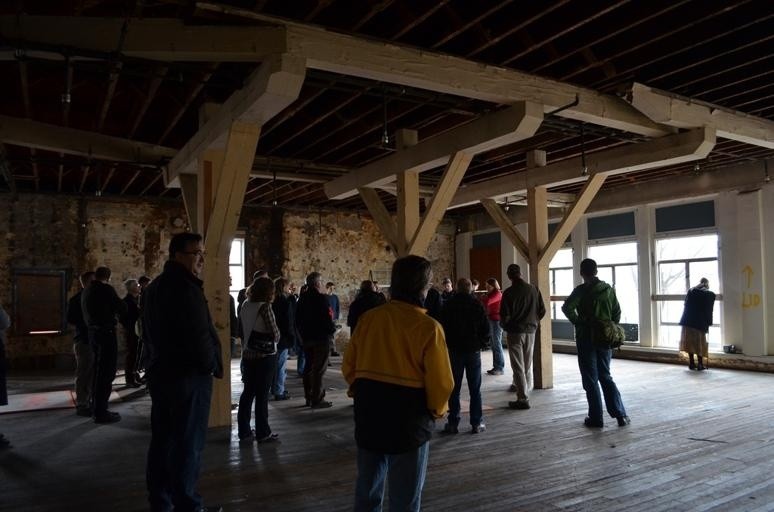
[579,122,589,176]
[60,57,73,104]
[763,154,771,185]
[381,82,394,144]
[269,156,282,207]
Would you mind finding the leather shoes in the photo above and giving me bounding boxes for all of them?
[617,416,631,426]
[585,416,604,428]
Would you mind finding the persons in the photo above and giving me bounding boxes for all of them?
[678,277,717,371]
[227,270,339,408]
[236,277,280,442]
[561,260,630,428]
[340,254,455,511]
[138,234,224,511]
[347,280,387,338]
[425,276,491,433]
[66,266,151,424]
[499,263,546,409]
[481,278,506,373]
[0,305,13,446]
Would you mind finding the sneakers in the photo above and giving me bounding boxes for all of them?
[508,400,530,409]
[94,410,122,424]
[472,423,486,433]
[689,363,705,370]
[274,394,292,401]
[239,429,279,443]
[306,396,332,409]
[76,408,94,418]
[126,377,145,388]
[444,423,458,432]
[487,368,504,375]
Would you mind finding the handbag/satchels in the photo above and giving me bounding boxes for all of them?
[598,320,625,349]
[247,330,275,353]
